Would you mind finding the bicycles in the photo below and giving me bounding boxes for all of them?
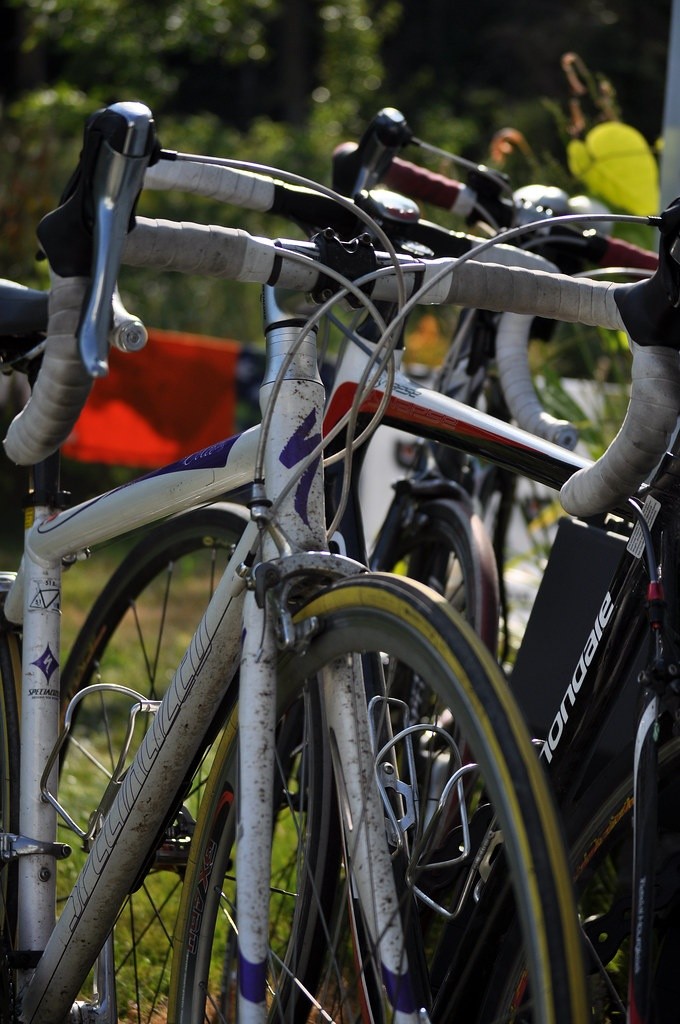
[0,81,680,1024]
